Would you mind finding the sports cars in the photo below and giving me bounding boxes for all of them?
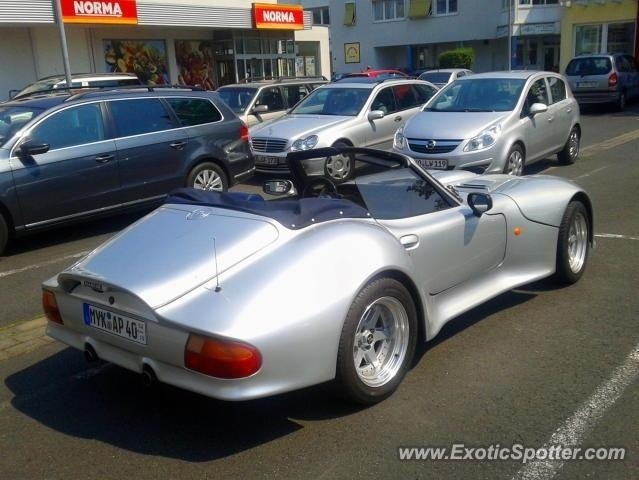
[40,145,595,407]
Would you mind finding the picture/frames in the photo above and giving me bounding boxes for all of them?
[344,42,360,64]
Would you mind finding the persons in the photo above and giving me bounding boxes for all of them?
[371,97,387,114]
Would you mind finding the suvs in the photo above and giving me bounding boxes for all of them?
[564,49,639,113]
